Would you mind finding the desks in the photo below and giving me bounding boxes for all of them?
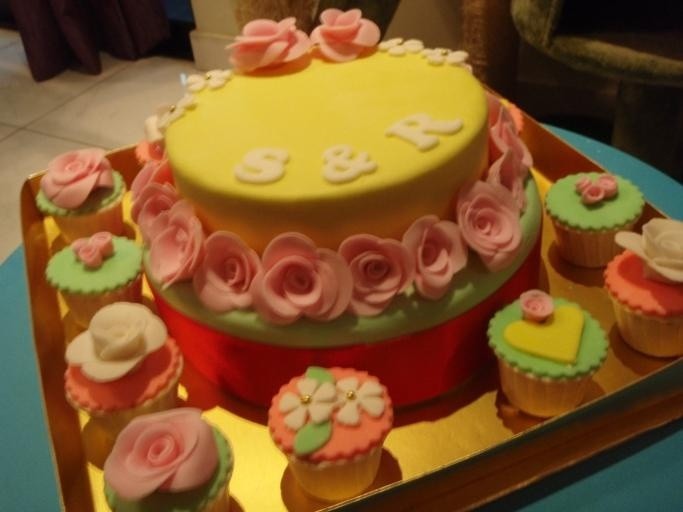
[0,124,683,512]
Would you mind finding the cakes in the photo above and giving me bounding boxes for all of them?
[269,364,394,501]
[63,301,185,434]
[544,169,645,269]
[34,147,127,244]
[485,289,609,418]
[102,407,234,512]
[602,218,683,362]
[129,7,546,412]
[45,230,145,328]
